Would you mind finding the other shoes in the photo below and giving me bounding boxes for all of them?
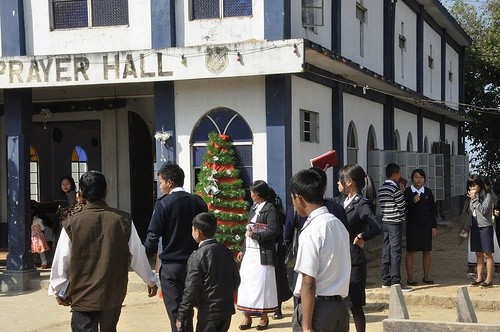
[470,279,484,287]
[479,282,493,288]
[272,312,283,320]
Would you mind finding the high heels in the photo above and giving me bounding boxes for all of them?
[256,314,270,330]
[407,278,418,285]
[423,278,434,283]
[239,317,253,330]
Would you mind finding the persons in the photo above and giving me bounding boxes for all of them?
[174,212,240,332]
[144,161,209,332]
[237,164,378,332]
[30,177,76,268]
[457,174,500,288]
[377,162,438,288]
[49,170,158,332]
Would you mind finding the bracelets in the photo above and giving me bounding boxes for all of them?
[357,235,364,241]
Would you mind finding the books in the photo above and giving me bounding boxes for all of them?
[397,174,409,190]
[246,223,269,234]
[309,150,338,169]
[57,276,71,297]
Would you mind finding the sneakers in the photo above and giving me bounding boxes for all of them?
[395,281,413,291]
[382,282,392,289]
